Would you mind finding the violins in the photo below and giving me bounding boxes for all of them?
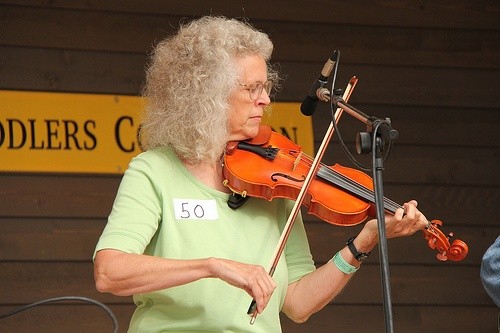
[222,124,468,262]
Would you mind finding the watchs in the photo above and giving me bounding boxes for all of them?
[346,234,372,262]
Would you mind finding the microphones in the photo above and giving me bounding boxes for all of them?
[300,50,338,116]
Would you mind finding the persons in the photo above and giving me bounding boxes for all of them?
[480,235,500,308]
[90,13,429,333]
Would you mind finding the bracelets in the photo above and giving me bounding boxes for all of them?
[333,251,360,275]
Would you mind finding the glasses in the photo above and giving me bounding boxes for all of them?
[239,80,273,101]
